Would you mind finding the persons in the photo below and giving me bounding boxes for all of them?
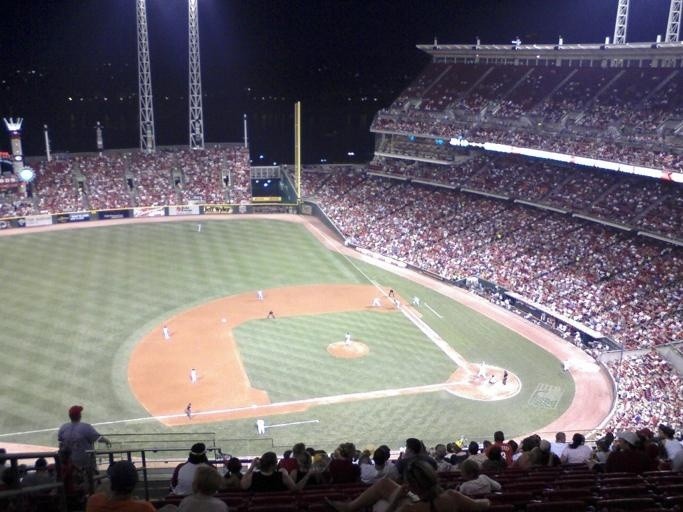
[185,403,193,421]
[388,288,394,298]
[0,142,249,218]
[257,288,263,300]
[189,368,197,384]
[371,296,382,306]
[467,285,683,423]
[256,417,265,434]
[287,62,682,283]
[266,309,275,319]
[163,323,170,341]
[0,422,683,512]
[412,296,420,307]
[343,331,350,344]
[57,405,111,482]
[393,298,401,310]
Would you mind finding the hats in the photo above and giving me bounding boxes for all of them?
[68,404,83,419]
[106,460,139,482]
[191,443,207,456]
[614,429,643,447]
